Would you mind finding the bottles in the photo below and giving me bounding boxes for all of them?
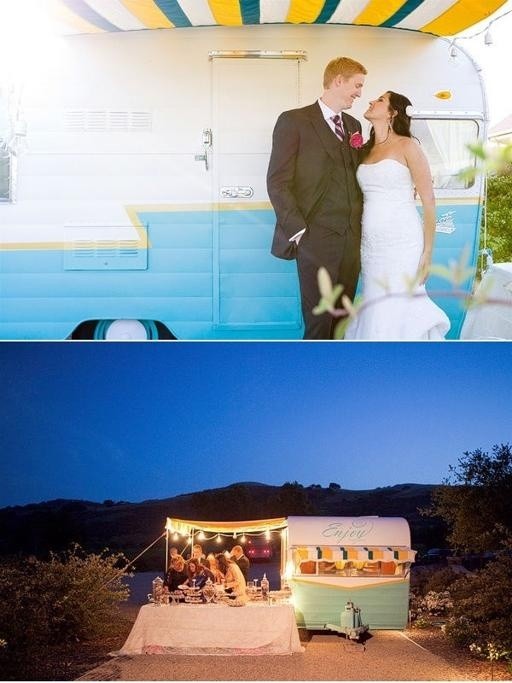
[261,573,269,601]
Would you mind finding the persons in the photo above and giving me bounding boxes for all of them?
[348,87,448,341]
[265,49,371,339]
[163,541,253,602]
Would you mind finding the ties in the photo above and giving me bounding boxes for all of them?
[330,115,345,142]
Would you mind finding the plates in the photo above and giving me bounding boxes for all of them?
[177,585,190,590]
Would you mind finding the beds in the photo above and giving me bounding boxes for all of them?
[104,599,307,658]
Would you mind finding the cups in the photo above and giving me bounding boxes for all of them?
[247,581,254,588]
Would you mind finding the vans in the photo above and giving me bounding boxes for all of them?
[0,20,489,345]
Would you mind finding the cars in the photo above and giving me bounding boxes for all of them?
[415,546,498,566]
[246,538,274,562]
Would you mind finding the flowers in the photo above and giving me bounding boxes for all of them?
[348,124,364,151]
